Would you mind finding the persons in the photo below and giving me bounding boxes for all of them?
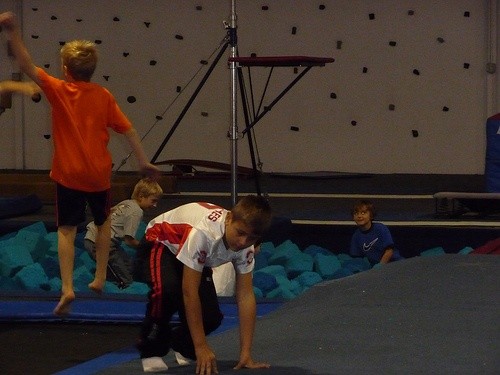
[0,80,42,103]
[84,176,164,285]
[0,13,161,315]
[132,195,273,375]
[350,200,400,267]
